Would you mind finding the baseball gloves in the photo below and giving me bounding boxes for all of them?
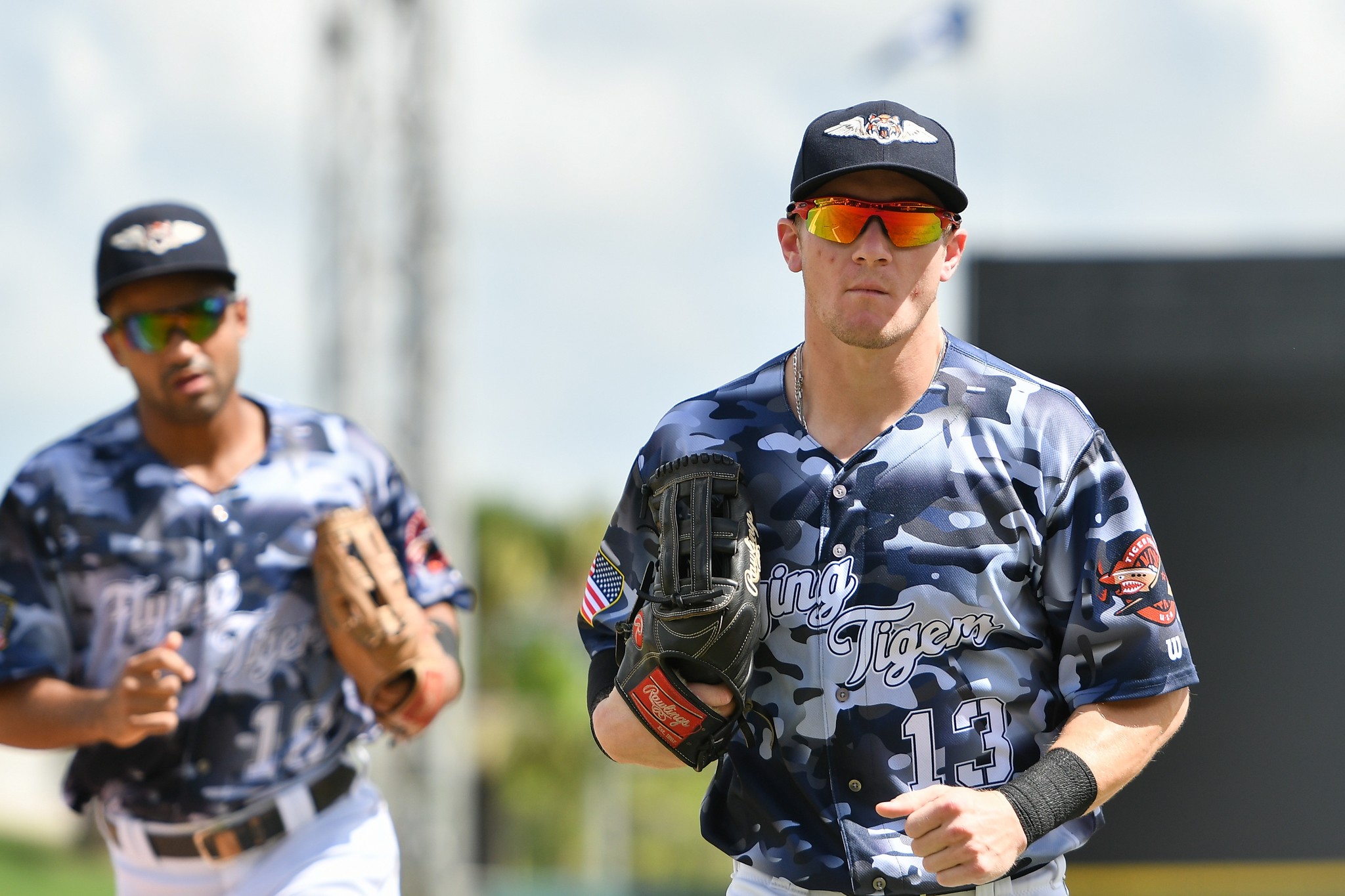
[312,507,443,742]
[612,452,762,774]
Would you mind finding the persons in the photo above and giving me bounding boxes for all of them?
[576,99,1200,896]
[0,200,476,896]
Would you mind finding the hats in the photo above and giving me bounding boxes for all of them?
[97,203,236,312]
[793,101,968,214]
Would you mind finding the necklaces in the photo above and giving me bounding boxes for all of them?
[792,329,947,434]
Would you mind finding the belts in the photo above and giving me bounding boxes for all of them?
[147,763,354,859]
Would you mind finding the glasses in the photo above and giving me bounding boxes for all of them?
[119,297,233,357]
[793,196,963,250]
